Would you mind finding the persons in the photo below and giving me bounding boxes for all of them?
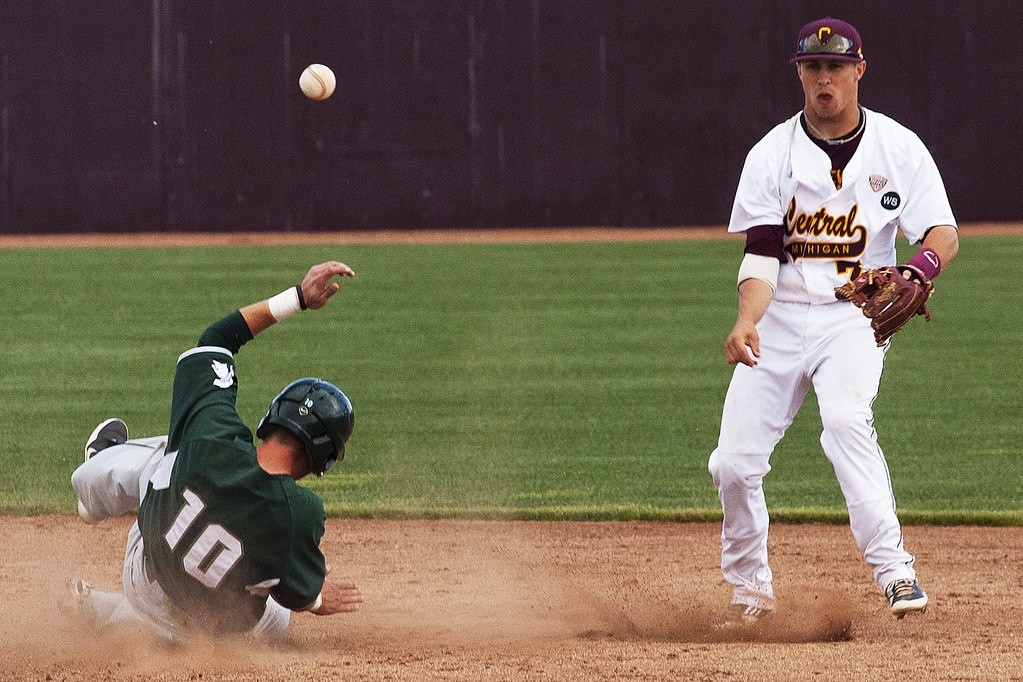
[66,258,365,648]
[707,17,961,632]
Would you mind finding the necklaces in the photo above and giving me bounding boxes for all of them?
[803,103,866,146]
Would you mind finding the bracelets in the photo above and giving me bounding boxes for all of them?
[907,247,942,281]
[268,286,302,324]
[296,285,307,311]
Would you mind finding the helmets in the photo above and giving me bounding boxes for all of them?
[256,376,355,478]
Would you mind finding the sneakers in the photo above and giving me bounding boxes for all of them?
[885,579,928,615]
[76,580,91,606]
[78,418,128,524]
[712,604,773,633]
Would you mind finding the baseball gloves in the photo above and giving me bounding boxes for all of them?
[834,264,936,347]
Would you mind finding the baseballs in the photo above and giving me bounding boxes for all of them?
[299,63,338,102]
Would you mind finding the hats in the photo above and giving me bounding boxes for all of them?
[789,16,863,63]
